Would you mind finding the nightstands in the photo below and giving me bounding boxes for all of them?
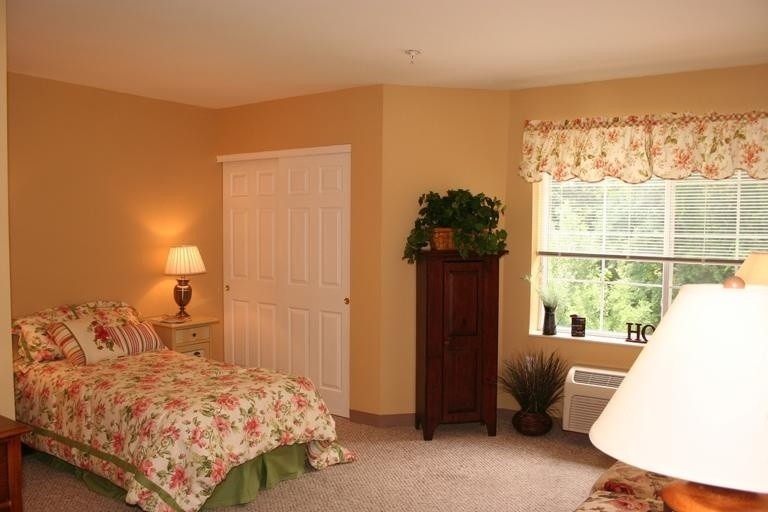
[143,313,220,360]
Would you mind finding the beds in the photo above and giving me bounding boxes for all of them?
[10,295,355,512]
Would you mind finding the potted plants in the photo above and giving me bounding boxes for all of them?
[402,187,507,264]
[495,345,567,435]
[521,272,558,336]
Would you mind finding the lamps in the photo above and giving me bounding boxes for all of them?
[586,277,768,512]
[163,244,206,317]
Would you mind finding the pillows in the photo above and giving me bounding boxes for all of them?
[70,299,144,357]
[105,320,166,355]
[43,312,126,369]
[10,302,79,365]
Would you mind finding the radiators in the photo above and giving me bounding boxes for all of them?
[559,362,629,436]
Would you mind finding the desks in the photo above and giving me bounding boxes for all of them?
[0,411,36,512]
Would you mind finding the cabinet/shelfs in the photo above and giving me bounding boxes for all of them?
[412,250,500,440]
